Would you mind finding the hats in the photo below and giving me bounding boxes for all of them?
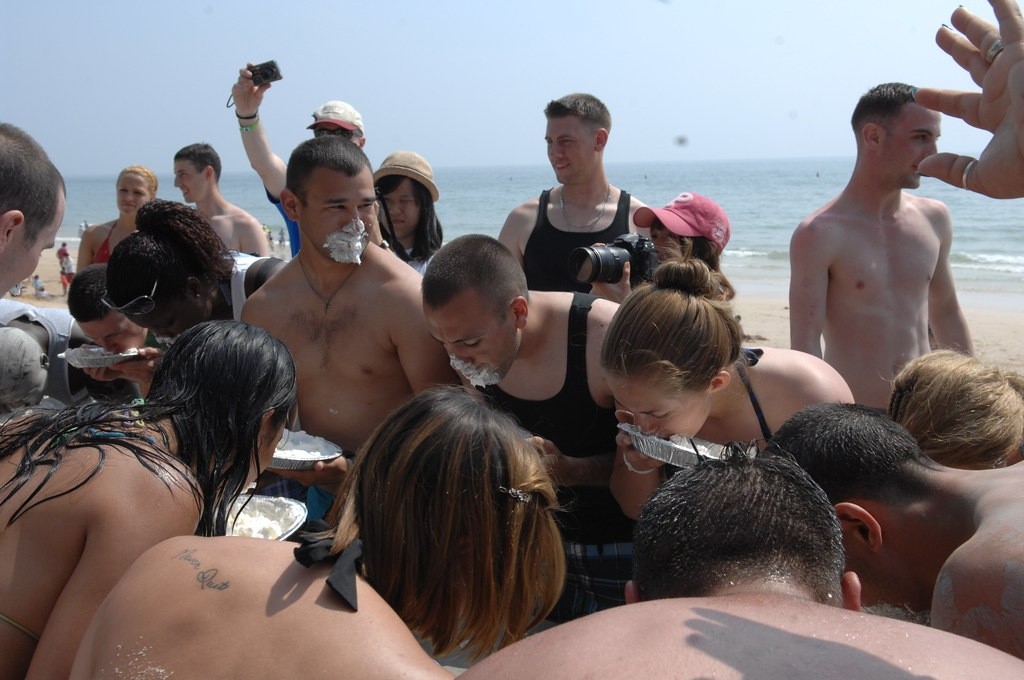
[306,99,364,134]
[633,192,730,254]
[373,152,440,204]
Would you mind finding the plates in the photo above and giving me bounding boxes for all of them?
[617,422,732,469]
[57,347,138,368]
[268,432,342,470]
[203,494,308,542]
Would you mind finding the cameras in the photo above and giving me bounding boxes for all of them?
[567,233,657,291]
[247,59,283,86]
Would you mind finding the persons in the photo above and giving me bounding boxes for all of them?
[0,123,570,680]
[788,81,975,411]
[912,0,1024,200]
[231,62,366,260]
[450,235,1024,680]
[497,92,732,304]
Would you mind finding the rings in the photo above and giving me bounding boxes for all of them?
[962,159,977,190]
[986,35,1003,64]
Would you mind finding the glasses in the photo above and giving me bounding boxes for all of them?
[312,128,361,141]
[100,276,161,316]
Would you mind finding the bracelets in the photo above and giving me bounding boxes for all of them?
[235,108,258,120]
[238,118,259,132]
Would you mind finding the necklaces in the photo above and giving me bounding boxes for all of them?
[299,249,365,316]
[559,184,610,227]
